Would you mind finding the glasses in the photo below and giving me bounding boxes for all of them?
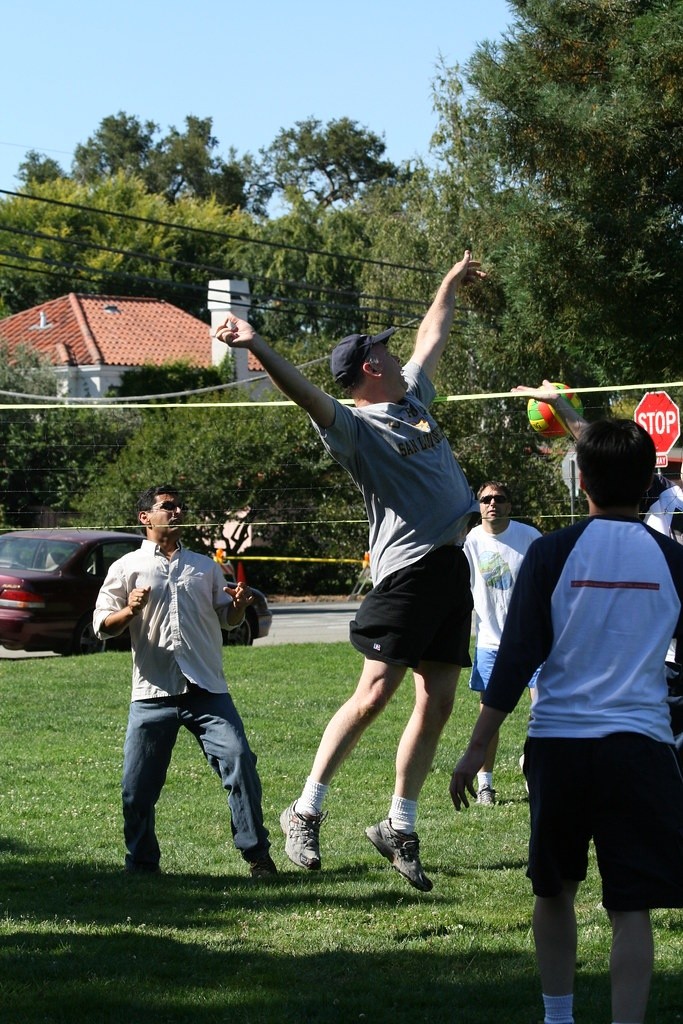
[479,495,507,504]
[152,501,184,511]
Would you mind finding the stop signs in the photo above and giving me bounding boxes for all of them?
[633,391,681,454]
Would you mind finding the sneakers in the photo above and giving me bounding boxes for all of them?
[363,818,434,892]
[280,799,330,870]
[251,854,278,880]
[477,785,495,808]
[519,754,530,795]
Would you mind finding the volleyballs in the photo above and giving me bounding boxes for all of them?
[526,382,583,439]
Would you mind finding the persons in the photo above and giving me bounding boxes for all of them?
[458,479,552,809]
[450,413,683,1024]
[93,483,282,889]
[510,378,683,771]
[210,246,486,892]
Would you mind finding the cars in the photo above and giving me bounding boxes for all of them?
[0,527,276,655]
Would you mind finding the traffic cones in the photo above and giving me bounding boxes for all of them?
[235,561,247,585]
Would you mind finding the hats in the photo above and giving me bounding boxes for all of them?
[331,327,394,388]
